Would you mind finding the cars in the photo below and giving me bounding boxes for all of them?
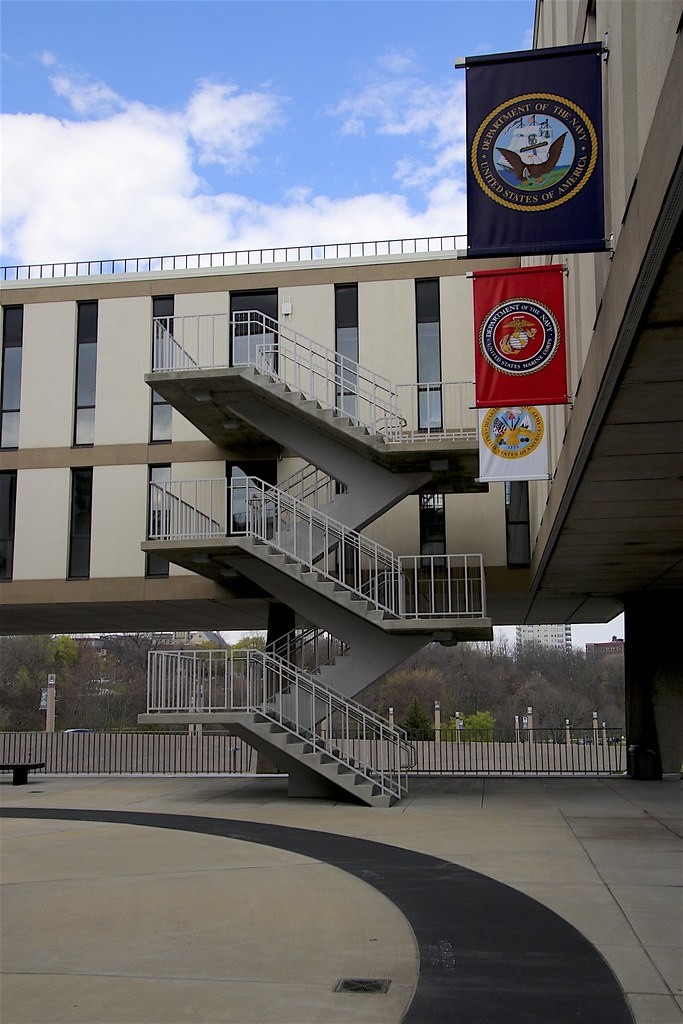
[64,728,89,732]
[577,739,593,745]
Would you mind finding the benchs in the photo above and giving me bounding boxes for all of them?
[0,762,46,786]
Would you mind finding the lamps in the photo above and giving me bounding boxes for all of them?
[597,49,610,64]
